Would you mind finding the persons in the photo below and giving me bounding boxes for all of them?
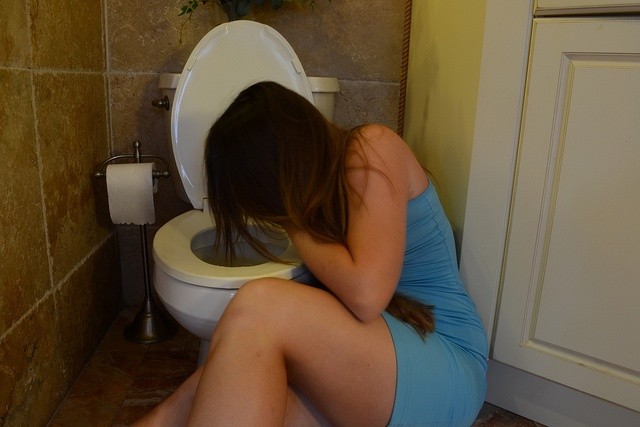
[129,82,488,427]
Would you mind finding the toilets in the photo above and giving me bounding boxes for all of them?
[153,20,338,385]
